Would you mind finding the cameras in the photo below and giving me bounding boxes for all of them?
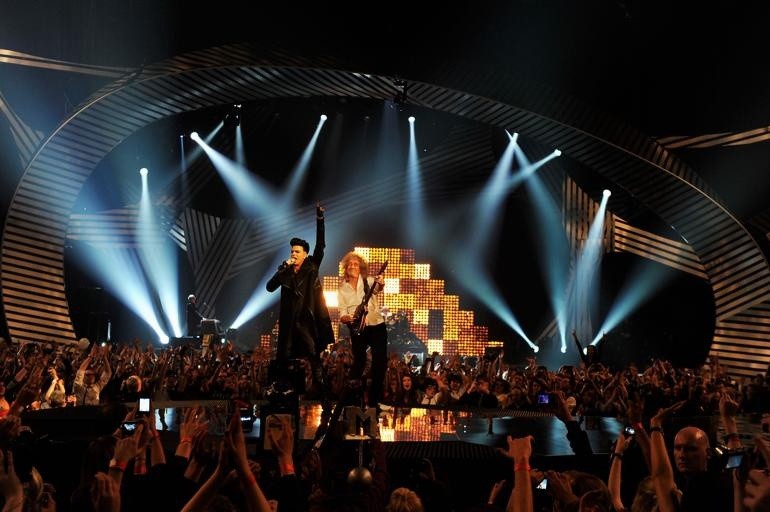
[712,442,754,471]
[624,425,637,440]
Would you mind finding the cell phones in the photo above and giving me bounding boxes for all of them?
[538,394,550,408]
[138,398,152,416]
[122,421,140,434]
[537,479,550,494]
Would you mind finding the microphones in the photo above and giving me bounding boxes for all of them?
[278,258,296,271]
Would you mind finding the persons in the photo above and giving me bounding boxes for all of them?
[264,200,337,402]
[334,250,388,408]
[185,293,210,334]
[1,329,770,512]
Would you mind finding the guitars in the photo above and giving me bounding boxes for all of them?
[351,260,388,335]
